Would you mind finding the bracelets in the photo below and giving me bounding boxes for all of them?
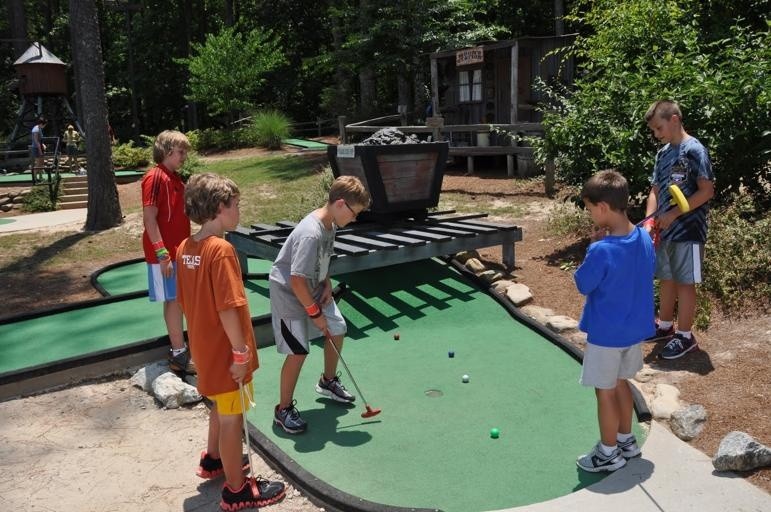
[304,303,322,318]
[151,241,170,264]
[231,345,251,364]
[643,218,655,226]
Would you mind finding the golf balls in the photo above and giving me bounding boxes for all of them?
[394,334,399,340]
[463,375,469,383]
[491,428,499,437]
[449,352,454,357]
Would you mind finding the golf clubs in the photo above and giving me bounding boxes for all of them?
[323,328,380,418]
[636,184,691,226]
[239,381,260,499]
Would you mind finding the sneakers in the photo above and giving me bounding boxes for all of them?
[316,372,356,404]
[642,318,698,360]
[198,449,252,479]
[219,476,286,511]
[165,346,199,376]
[34,177,45,185]
[576,434,641,473]
[273,403,308,435]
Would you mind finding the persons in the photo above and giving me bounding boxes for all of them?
[268,175,371,434]
[574,169,658,473]
[174,172,287,512]
[141,130,192,378]
[30,117,48,183]
[61,124,78,174]
[643,98,714,361]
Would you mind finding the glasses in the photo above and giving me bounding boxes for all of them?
[343,200,359,218]
